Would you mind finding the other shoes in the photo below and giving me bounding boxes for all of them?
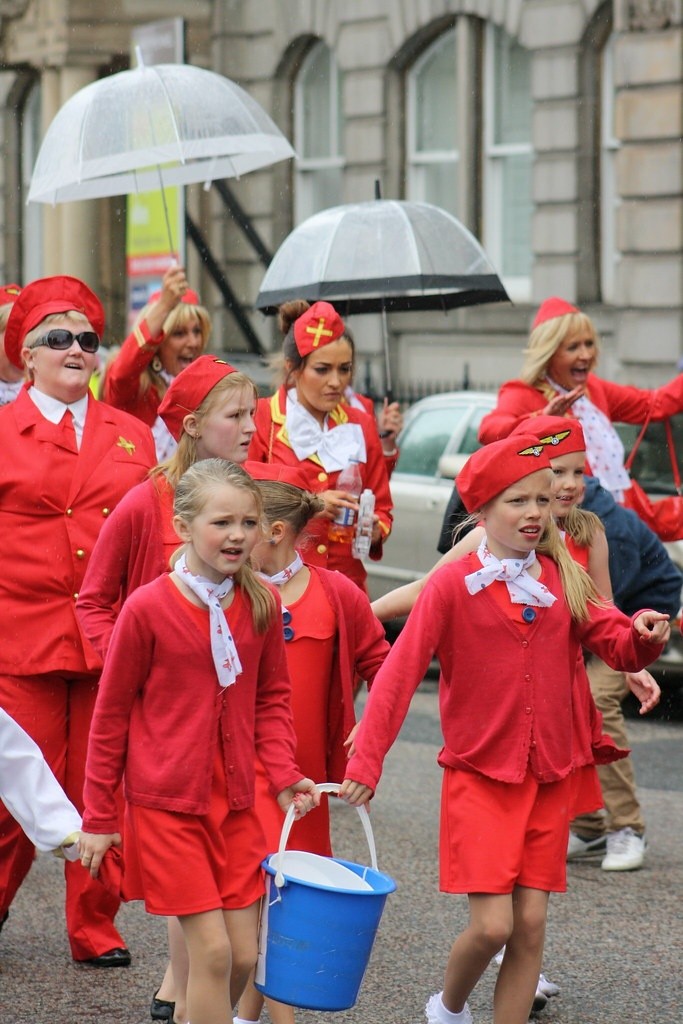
[150,989,175,1024]
[496,945,558,1012]
[90,948,132,966]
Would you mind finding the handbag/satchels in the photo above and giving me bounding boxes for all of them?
[613,387,683,541]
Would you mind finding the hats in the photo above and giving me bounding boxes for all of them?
[293,300,346,358]
[147,289,198,305]
[510,416,585,458]
[453,433,552,514]
[3,275,105,371]
[157,354,239,443]
[531,296,579,332]
[0,284,22,306]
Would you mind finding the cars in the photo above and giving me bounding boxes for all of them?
[361,389,683,672]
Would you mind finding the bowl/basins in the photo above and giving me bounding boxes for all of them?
[269,850,373,891]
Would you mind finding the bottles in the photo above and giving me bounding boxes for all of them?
[326,455,362,544]
[351,489,376,559]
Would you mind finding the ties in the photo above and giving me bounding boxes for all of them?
[58,409,77,449]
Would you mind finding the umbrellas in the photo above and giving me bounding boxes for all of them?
[255,178,510,437]
[25,46,296,274]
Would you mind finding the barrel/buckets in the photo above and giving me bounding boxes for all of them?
[254,782,397,1011]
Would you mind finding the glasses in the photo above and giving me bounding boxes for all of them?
[28,329,100,353]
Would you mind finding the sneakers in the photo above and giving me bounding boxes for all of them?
[567,830,606,859]
[602,827,647,871]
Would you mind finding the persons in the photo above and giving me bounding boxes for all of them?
[338,435,671,1024]
[80,457,321,1024]
[0,273,683,1023]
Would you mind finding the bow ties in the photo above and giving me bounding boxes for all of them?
[286,401,366,474]
